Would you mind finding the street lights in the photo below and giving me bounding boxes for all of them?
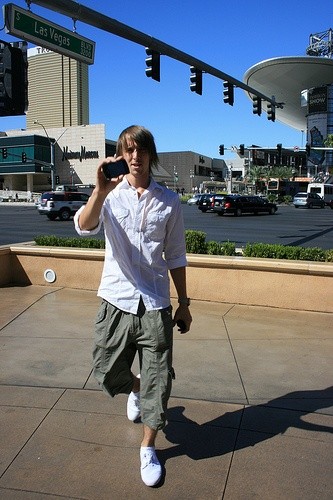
[33,120,71,186]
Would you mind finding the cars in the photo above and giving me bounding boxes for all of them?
[186,195,203,205]
[293,192,324,210]
[198,193,215,213]
[211,195,280,214]
[37,191,91,221]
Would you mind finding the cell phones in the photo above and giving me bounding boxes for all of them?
[102,159,130,180]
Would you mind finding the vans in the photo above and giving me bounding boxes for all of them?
[307,183,333,203]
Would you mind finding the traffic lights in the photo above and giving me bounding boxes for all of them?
[55,176,59,184]
[21,152,27,163]
[266,102,276,122]
[252,97,262,116]
[223,79,234,106]
[305,144,311,156]
[145,46,160,83]
[276,144,282,156]
[2,148,7,158]
[188,65,203,96]
[219,144,224,155]
[239,144,244,154]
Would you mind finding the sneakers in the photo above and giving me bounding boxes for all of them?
[139,440,163,487]
[126,373,141,421]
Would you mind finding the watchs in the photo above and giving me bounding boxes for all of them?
[178,297,190,307]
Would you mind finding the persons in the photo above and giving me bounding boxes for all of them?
[176,186,210,196]
[74,126,192,488]
